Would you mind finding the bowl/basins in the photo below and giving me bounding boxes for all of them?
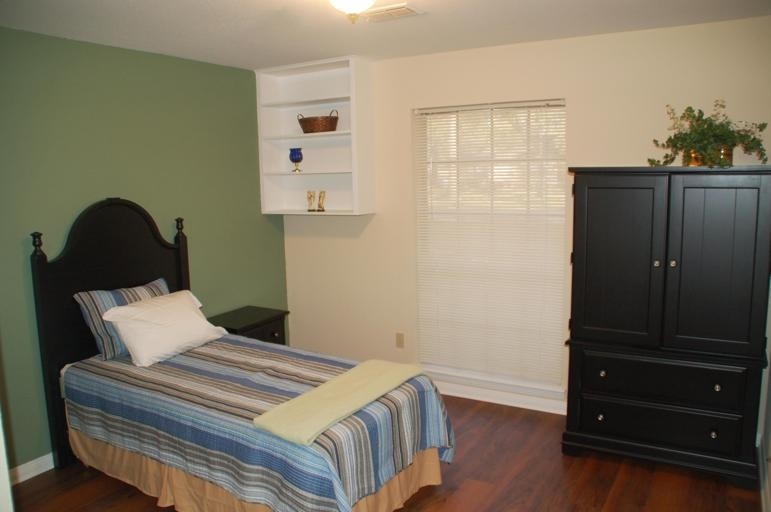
[296,109,338,133]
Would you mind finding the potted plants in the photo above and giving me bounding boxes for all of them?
[648,96,768,168]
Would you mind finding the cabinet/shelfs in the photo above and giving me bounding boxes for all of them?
[253,54,372,216]
[560,166,771,488]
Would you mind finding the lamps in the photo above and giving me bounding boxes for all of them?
[329,0,375,26]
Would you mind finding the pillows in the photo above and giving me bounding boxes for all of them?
[71,277,171,361]
[101,289,228,369]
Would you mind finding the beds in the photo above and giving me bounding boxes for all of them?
[27,197,456,512]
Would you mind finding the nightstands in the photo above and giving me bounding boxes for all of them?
[205,305,289,345]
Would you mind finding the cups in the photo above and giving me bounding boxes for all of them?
[289,148,303,172]
